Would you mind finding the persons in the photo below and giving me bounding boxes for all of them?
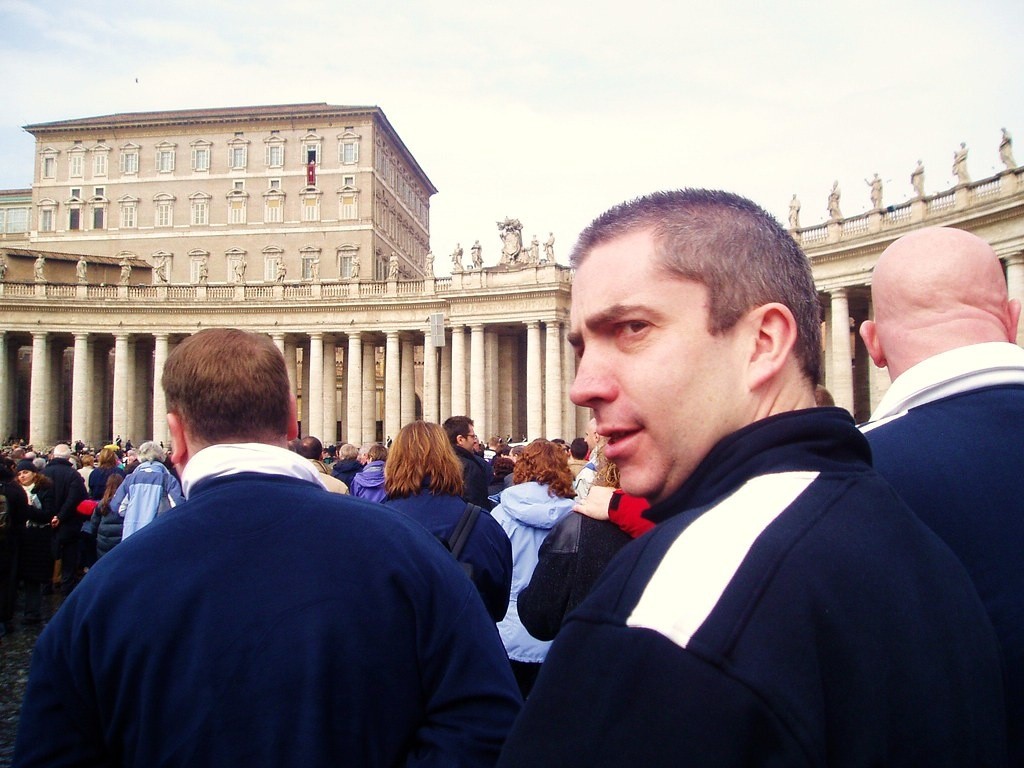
[854,226,1024,768]
[11,329,523,768]
[0,125,1016,283]
[0,385,836,698]
[494,188,1007,768]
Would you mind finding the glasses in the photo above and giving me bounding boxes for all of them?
[462,433,477,439]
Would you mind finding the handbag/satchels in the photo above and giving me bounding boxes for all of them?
[156,474,172,520]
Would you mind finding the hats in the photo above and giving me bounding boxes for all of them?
[17,460,36,472]
[104,445,120,452]
